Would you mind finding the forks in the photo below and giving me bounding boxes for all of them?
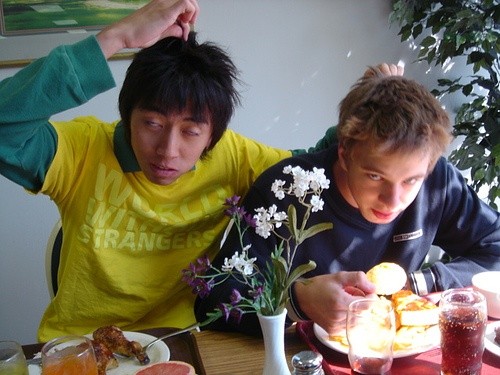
[113,323,200,359]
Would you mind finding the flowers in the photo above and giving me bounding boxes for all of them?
[194,163,331,321]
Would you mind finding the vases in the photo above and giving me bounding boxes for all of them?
[257,308,293,375]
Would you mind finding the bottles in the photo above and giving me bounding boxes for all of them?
[292,350,325,375]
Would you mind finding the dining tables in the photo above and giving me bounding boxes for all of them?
[188,322,308,375]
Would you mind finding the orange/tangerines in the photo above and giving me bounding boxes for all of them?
[134,360,196,375]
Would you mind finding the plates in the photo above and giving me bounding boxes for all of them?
[27,331,171,375]
[313,321,441,358]
[484,320,500,357]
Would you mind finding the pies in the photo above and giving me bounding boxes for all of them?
[389,289,442,351]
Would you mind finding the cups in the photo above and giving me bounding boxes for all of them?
[0,340,29,375]
[347,299,394,374]
[437,288,488,375]
[134,360,195,375]
[41,335,98,375]
[471,271,500,319]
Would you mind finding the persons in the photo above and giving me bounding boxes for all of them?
[0,0,404,346]
[193,64,500,338]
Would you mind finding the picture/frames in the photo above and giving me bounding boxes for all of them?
[0,0,194,69]
